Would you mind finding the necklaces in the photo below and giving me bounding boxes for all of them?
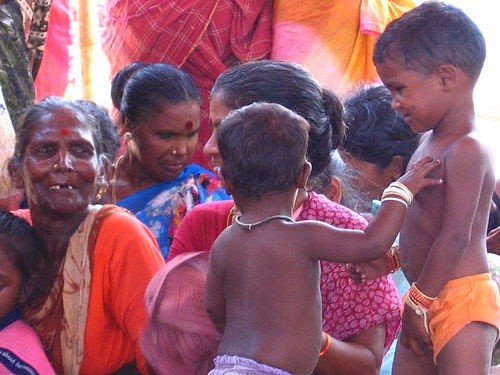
[235,215,296,231]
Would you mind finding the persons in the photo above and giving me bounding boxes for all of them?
[203,102,443,375]
[7,100,167,375]
[90,61,234,261]
[172,60,401,375]
[336,84,499,254]
[344,1,499,375]
[0,210,56,374]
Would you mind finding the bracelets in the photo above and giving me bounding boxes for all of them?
[385,244,401,274]
[403,282,438,333]
[380,181,414,208]
